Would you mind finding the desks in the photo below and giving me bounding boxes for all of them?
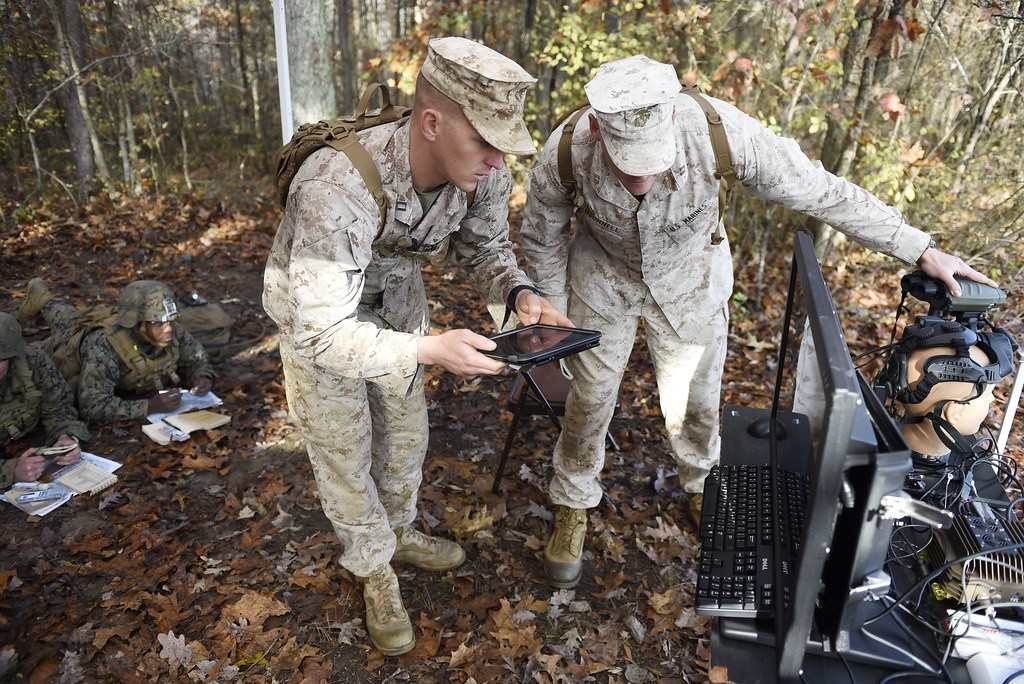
[707,402,1024,684]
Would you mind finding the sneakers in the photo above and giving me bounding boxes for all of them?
[16,277,55,321]
[543,505,587,589]
[687,493,703,529]
[363,563,415,656]
[389,525,466,577]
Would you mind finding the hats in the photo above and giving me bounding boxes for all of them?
[584,54,683,177]
[420,36,538,155]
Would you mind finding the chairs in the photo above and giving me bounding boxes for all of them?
[491,358,624,515]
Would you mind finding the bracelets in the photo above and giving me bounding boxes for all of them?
[507,285,543,316]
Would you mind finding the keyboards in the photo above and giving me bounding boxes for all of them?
[777,464,812,610]
[695,463,776,619]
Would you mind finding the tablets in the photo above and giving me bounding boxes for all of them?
[475,324,602,364]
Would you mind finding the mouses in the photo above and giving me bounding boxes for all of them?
[775,418,787,441]
[747,418,770,438]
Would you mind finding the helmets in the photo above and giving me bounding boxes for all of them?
[118,280,180,328]
[0,312,26,360]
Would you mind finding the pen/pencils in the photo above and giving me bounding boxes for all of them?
[160,418,182,433]
[159,390,189,394]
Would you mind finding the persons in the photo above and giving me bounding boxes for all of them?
[0,312,91,491]
[888,316,995,456]
[262,36,576,656]
[17,278,219,423]
[520,54,998,588]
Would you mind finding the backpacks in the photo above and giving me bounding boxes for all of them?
[177,303,264,359]
[273,82,477,244]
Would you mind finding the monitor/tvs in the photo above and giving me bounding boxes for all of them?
[719,230,913,684]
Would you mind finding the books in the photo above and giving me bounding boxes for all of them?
[142,410,231,445]
[3,461,118,516]
[34,443,78,458]
